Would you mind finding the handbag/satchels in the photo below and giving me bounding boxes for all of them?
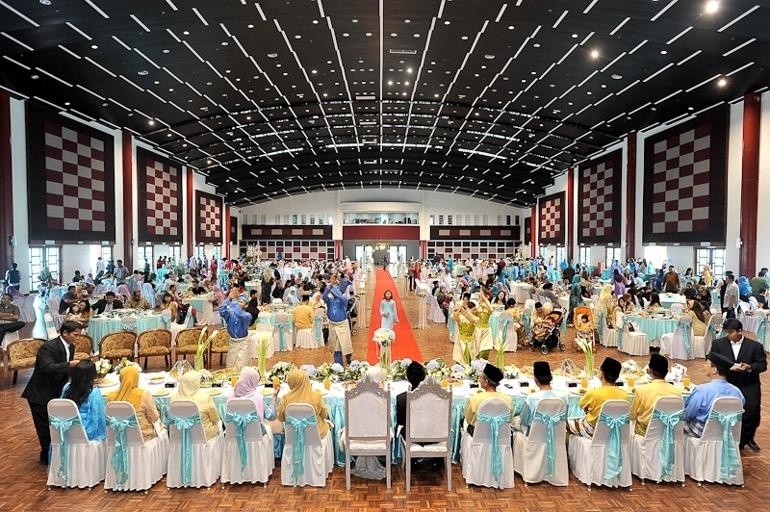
[176,303,188,323]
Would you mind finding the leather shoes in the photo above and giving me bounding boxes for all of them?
[747,440,760,450]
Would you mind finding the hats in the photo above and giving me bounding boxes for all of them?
[602,357,621,375]
[484,363,503,382]
[650,354,668,371]
[707,351,734,369]
[238,296,250,305]
[535,302,542,308]
[534,362,551,376]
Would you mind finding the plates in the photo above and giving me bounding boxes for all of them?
[149,375,166,380]
[445,381,464,387]
[522,388,536,394]
[149,381,164,384]
[261,388,274,395]
[679,386,692,395]
[207,390,223,395]
[337,381,355,391]
[101,389,112,396]
[569,387,591,395]
[625,386,636,396]
[151,390,169,397]
[314,387,327,395]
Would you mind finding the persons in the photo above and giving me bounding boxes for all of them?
[406,254,769,361]
[394,361,444,472]
[683,352,746,438]
[19,321,84,465]
[464,362,515,437]
[383,255,388,270]
[226,365,280,437]
[106,366,160,441]
[511,362,568,435]
[2,256,367,363]
[378,289,399,329]
[630,354,682,438]
[47,359,106,465]
[277,368,330,440]
[170,371,220,442]
[566,357,629,445]
[712,319,767,453]
[350,366,391,481]
